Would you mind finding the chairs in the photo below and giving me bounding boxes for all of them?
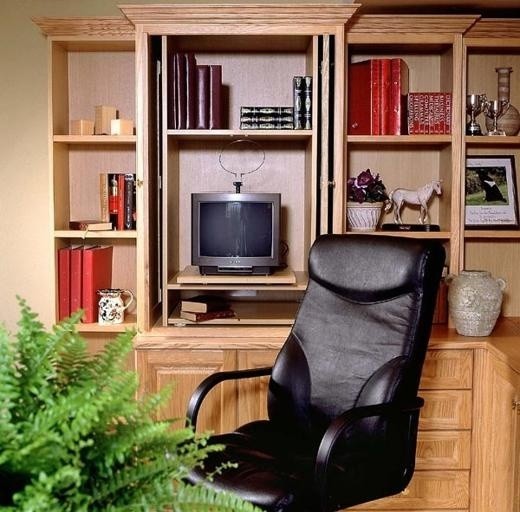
[172,233,446,511]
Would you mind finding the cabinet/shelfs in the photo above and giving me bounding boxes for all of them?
[60,336,135,402]
[346,349,473,511]
[30,15,136,332]
[117,4,361,338]
[132,342,284,512]
[459,17,520,319]
[344,14,480,330]
[471,348,520,512]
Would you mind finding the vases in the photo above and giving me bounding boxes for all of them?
[346,201,385,232]
[443,270,507,337]
[485,66,520,137]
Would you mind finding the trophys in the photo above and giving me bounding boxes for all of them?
[466,92,489,136]
[483,97,511,136]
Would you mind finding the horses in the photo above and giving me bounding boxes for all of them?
[384,178,444,224]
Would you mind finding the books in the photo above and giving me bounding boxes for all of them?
[177,309,237,323]
[181,293,231,314]
[170,46,452,136]
[57,168,138,324]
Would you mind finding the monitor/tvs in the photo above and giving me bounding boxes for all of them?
[190,192,281,276]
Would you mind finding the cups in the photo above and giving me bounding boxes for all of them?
[96,288,133,325]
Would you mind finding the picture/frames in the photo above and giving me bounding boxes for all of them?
[466,154,519,229]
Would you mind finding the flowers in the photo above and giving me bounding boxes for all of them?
[347,166,390,203]
